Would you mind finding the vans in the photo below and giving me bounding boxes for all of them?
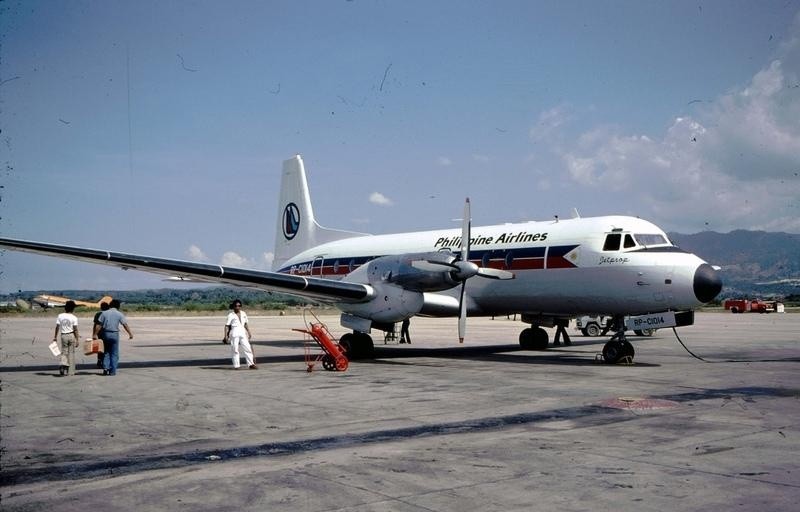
[576,315,656,337]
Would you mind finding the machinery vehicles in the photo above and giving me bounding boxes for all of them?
[725,296,775,313]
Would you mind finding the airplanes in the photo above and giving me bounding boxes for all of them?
[32,293,113,312]
[0,156,725,367]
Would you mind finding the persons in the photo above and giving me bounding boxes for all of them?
[385,330,396,341]
[53,301,79,375]
[224,299,258,369]
[93,302,109,369]
[92,299,133,376]
[400,318,411,344]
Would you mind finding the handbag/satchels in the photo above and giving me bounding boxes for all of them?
[84,337,105,354]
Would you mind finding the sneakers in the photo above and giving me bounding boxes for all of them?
[97,359,116,375]
[61,365,65,375]
[250,365,256,369]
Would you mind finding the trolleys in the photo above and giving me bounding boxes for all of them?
[291,308,349,373]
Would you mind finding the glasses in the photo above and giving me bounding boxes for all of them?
[235,305,241,307]
